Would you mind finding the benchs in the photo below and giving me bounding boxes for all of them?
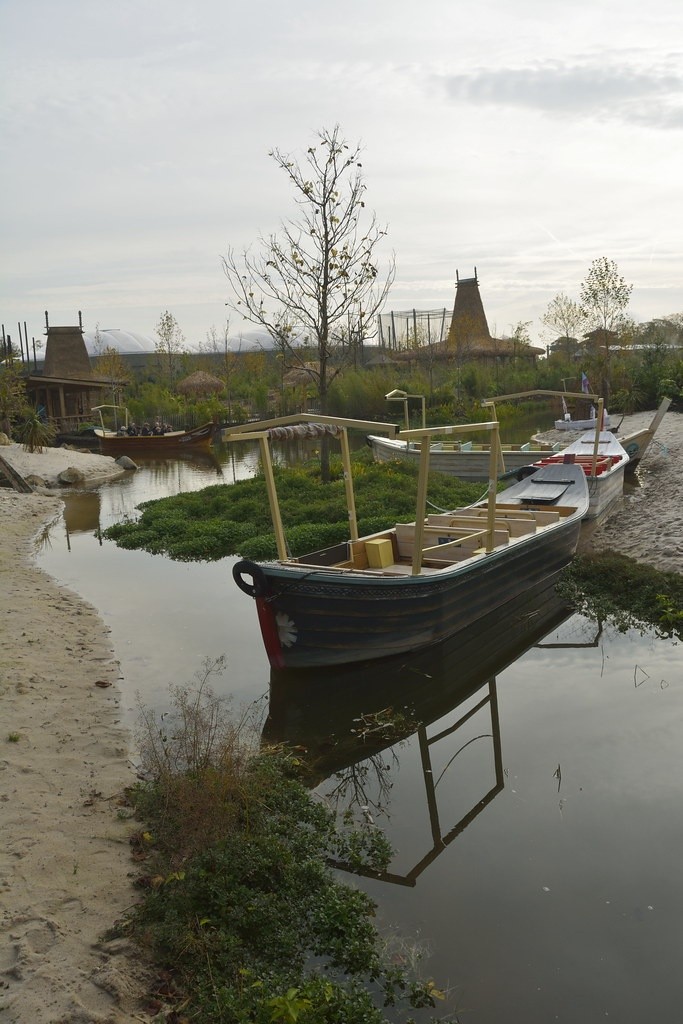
[533,454,623,475]
[395,508,566,566]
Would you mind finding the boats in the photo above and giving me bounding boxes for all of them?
[219,385,590,677]
[479,389,630,551]
[364,388,673,484]
[555,396,611,431]
[91,405,214,451]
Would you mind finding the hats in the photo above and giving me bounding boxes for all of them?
[144,423,150,426]
[121,426,127,431]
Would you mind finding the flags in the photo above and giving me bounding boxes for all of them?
[581,372,589,394]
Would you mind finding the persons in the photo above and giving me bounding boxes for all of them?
[127,423,138,436]
[117,426,129,437]
[142,423,153,436]
[38,401,47,421]
[152,422,164,435]
